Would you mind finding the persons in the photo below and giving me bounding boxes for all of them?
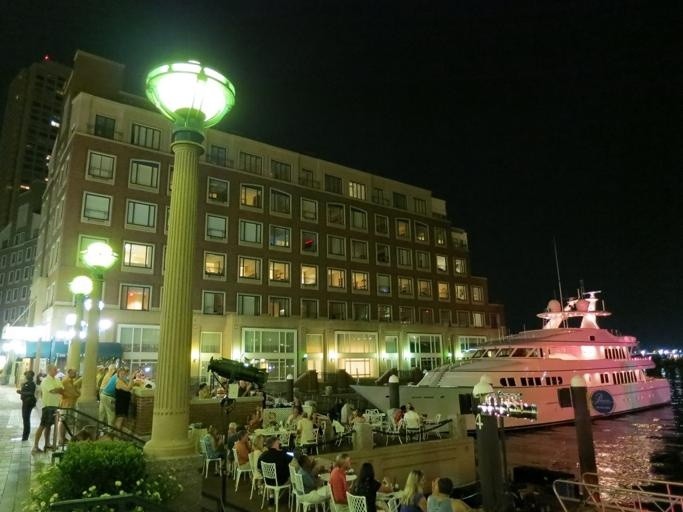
[17,362,154,454]
[197,379,473,512]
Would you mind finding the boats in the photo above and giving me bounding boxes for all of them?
[346,237,674,439]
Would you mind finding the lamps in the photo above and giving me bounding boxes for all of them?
[302,353,308,362]
[476,391,538,421]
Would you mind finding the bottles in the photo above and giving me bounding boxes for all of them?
[393,479,400,491]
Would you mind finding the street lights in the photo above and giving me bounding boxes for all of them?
[62,272,95,377]
[131,57,238,458]
[79,239,119,403]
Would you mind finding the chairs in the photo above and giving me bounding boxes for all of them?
[189,393,481,512]
